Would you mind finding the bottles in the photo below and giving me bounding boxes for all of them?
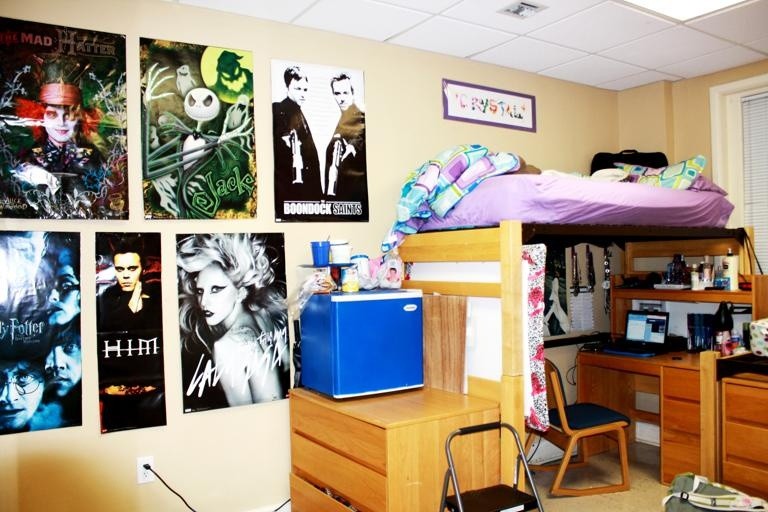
[689,254,722,291]
[714,327,741,358]
[681,264,690,285]
[381,245,402,289]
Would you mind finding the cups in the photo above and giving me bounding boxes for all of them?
[310,241,330,267]
[741,322,751,350]
[329,241,349,263]
[685,313,711,354]
[349,252,371,279]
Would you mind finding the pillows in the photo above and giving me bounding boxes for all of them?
[615,161,728,194]
[620,155,706,189]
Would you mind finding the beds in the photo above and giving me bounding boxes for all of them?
[396,219,756,494]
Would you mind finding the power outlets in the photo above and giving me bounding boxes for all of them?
[137,456,154,483]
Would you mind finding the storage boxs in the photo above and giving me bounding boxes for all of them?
[301,289,424,400]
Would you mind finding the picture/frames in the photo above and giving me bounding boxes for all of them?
[442,79,536,132]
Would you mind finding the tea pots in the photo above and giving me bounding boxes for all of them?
[712,301,737,331]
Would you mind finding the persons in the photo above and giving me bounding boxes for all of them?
[96,246,161,383]
[325,74,366,221]
[273,65,325,219]
[177,233,290,408]
[12,77,105,218]
[0,250,82,435]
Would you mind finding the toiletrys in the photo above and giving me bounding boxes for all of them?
[691,263,700,291]
[703,255,712,281]
[713,329,741,357]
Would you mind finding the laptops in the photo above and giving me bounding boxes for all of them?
[604,309,669,357]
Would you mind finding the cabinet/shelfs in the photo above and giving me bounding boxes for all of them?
[610,237,768,342]
[289,386,502,512]
[575,341,720,488]
[717,351,768,499]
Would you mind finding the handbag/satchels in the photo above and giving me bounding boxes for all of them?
[664,472,768,511]
[591,150,668,175]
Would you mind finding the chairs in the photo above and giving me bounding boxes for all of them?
[525,357,631,496]
[440,422,543,512]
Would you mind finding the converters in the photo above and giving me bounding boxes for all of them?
[584,343,595,349]
